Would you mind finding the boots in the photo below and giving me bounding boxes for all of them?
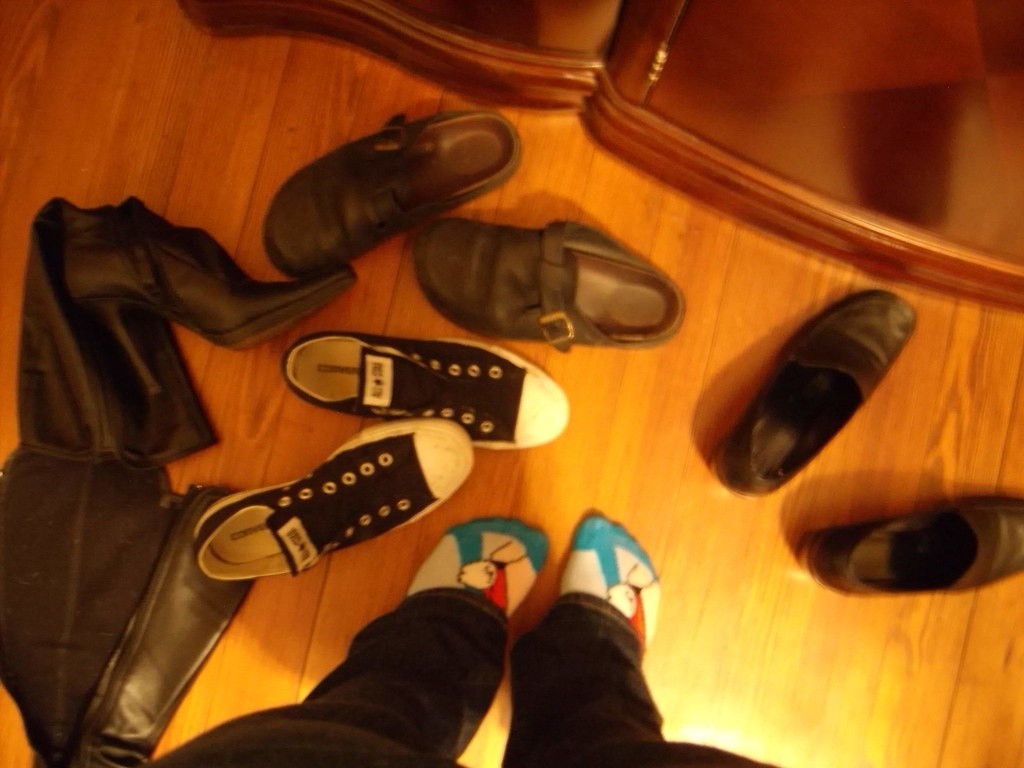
[17,196,355,464]
[0,450,256,768]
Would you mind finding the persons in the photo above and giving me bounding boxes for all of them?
[151,513,785,768]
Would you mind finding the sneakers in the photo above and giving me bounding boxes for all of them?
[283,331,568,449]
[191,419,474,583]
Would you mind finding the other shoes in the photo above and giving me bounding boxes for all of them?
[711,289,918,498]
[795,496,1024,596]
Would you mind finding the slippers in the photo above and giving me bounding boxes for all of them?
[414,217,684,353]
[263,109,521,278]
